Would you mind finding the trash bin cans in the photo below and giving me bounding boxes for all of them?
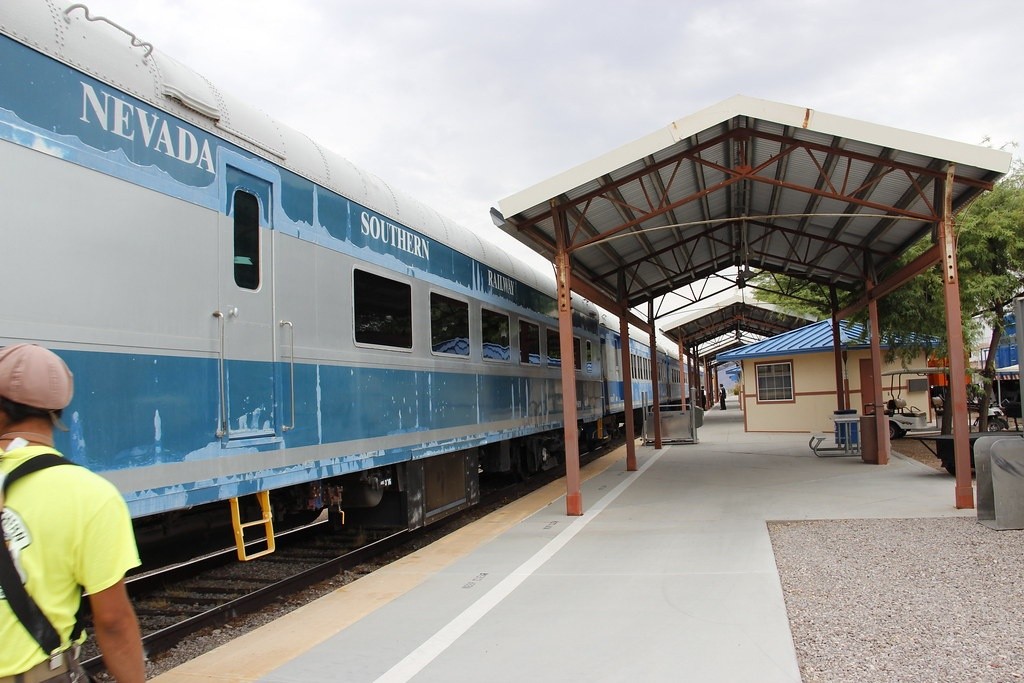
[833,409,860,445]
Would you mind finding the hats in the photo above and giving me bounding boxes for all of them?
[0,343,74,410]
[720,384,724,387]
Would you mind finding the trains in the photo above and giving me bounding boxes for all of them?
[0,0,706,562]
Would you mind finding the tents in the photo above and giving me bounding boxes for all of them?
[659,294,818,412]
[490,94,1014,519]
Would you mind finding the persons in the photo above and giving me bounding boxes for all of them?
[0,342,151,683]
[719,383,727,411]
[700,385,708,411]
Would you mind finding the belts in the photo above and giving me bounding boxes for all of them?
[0,646,79,683]
